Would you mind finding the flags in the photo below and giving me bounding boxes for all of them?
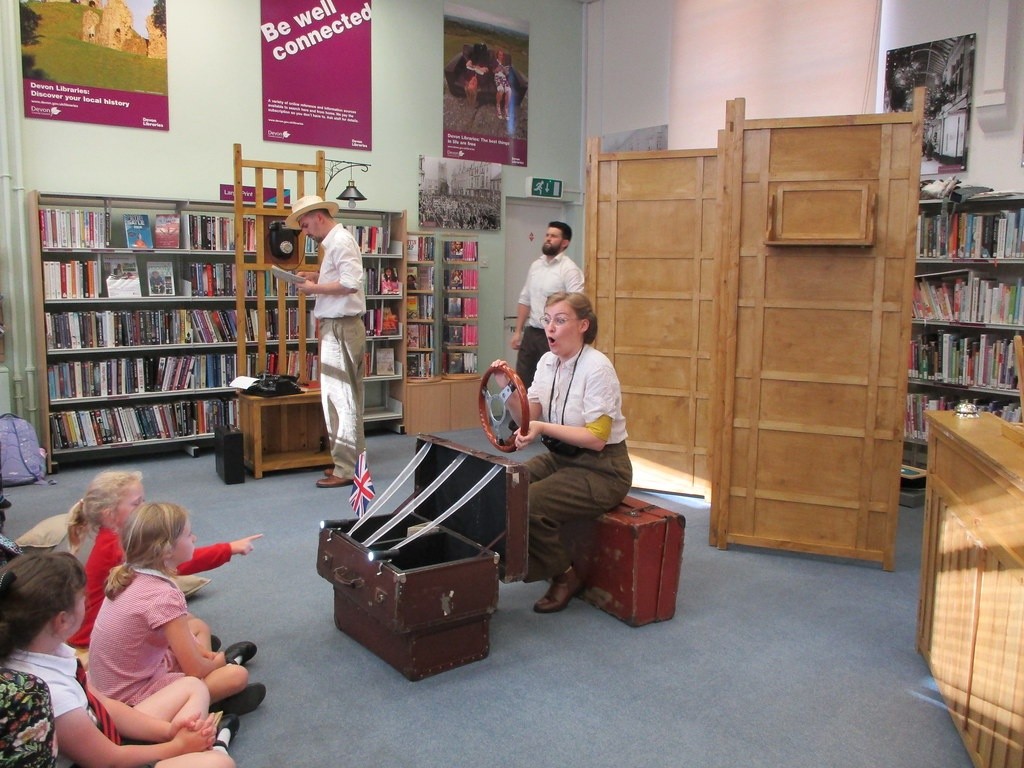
[348,452,376,518]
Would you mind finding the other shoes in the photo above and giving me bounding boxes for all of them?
[224,641,257,666]
[213,714,240,749]
[210,634,222,652]
[208,683,267,717]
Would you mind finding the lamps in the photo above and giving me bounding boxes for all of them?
[324,159,371,208]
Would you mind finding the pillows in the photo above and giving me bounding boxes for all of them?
[15,514,68,549]
[177,575,212,598]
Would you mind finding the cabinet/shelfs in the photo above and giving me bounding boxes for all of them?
[25,191,407,474]
[906,194,1024,465]
[408,231,481,383]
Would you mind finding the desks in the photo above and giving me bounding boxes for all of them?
[407,379,482,436]
[235,391,334,479]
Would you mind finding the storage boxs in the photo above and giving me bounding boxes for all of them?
[317,432,530,682]
[570,495,687,628]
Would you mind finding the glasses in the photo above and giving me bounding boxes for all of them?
[539,316,579,327]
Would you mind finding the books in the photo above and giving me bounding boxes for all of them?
[342,222,403,380]
[902,205,1024,443]
[38,207,321,454]
[405,231,478,381]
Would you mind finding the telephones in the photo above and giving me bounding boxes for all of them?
[268,220,302,259]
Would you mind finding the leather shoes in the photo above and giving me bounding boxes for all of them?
[324,469,333,475]
[533,568,586,614]
[316,474,354,487]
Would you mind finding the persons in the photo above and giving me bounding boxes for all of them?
[493,49,512,122]
[509,220,586,434]
[284,194,373,489]
[0,470,265,768]
[419,192,501,230]
[489,290,634,613]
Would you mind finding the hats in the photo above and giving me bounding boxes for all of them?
[287,195,339,228]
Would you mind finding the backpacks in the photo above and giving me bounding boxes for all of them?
[0,412,58,485]
